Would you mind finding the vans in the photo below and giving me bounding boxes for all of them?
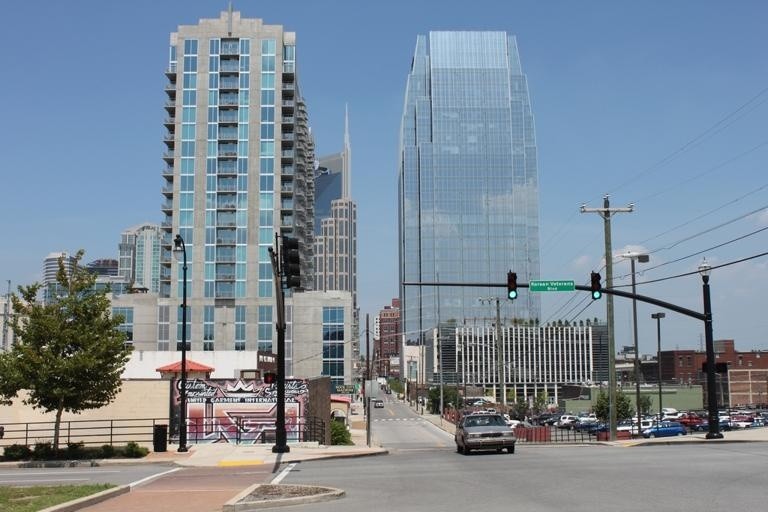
[463,397,487,405]
[470,408,522,429]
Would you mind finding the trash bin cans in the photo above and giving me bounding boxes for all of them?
[153,424,168,452]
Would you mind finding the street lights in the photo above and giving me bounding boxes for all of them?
[699,257,723,439]
[172,233,190,452]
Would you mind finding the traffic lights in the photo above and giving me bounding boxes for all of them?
[282,236,301,289]
[590,271,602,300]
[506,270,518,300]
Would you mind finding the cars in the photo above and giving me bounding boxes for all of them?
[374,400,384,408]
[473,401,488,407]
[371,396,377,402]
[529,403,768,438]
[454,413,517,455]
